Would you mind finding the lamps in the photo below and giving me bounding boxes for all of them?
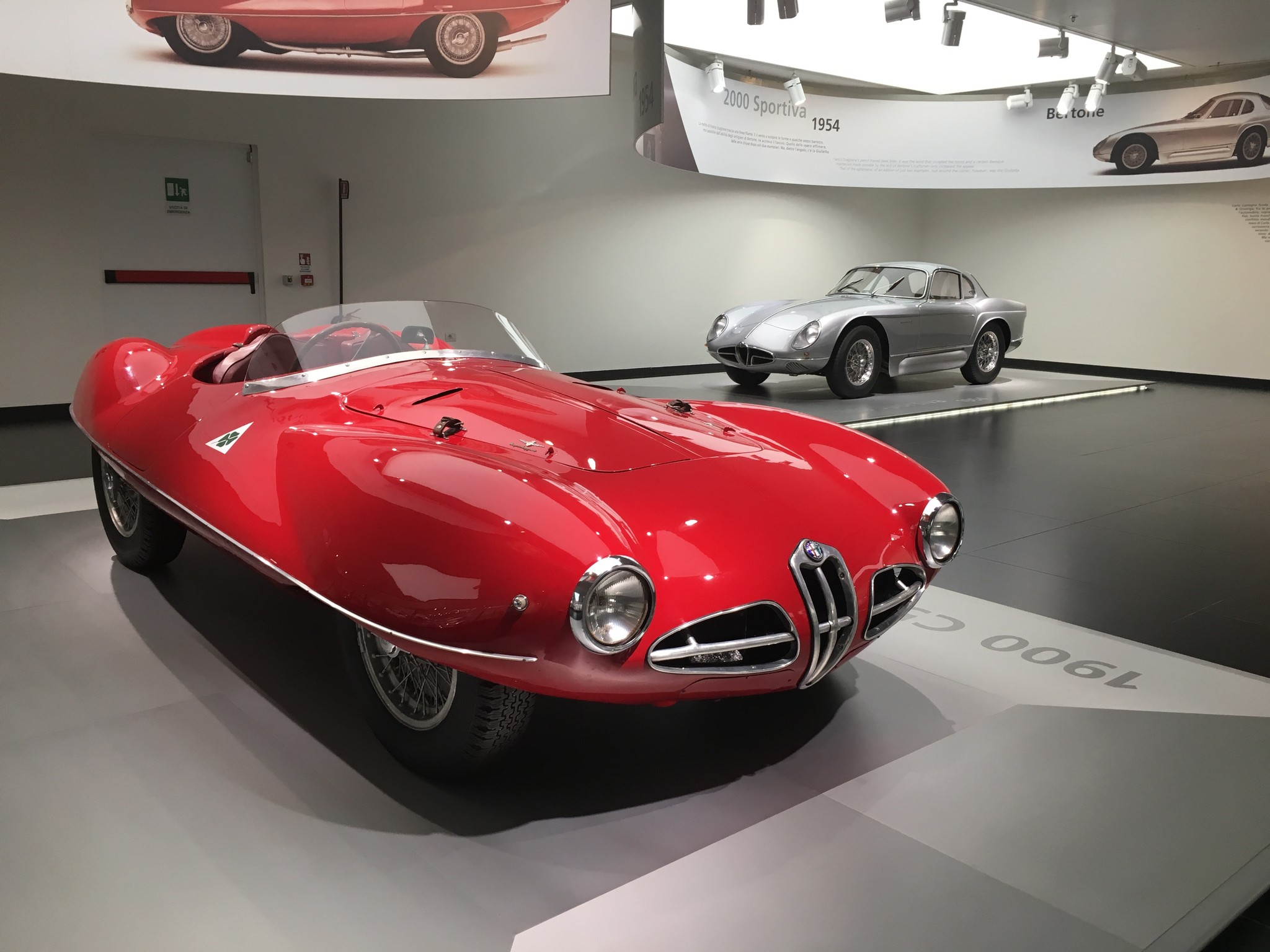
[1056,81,1080,115]
[777,0,799,20]
[705,54,726,95]
[1095,44,1124,85]
[1006,86,1033,110]
[1122,49,1146,81]
[747,0,766,25]
[1037,27,1069,59]
[941,0,967,47]
[1084,81,1107,112]
[783,70,806,105]
[885,0,920,24]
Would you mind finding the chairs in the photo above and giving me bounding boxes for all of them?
[340,323,417,358]
[886,289,907,297]
[213,331,329,384]
[915,287,940,300]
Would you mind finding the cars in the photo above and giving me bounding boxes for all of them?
[128,1,573,78]
[1094,93,1269,172]
[705,264,1027,399]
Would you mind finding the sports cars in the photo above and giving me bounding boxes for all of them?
[71,299,963,774]
